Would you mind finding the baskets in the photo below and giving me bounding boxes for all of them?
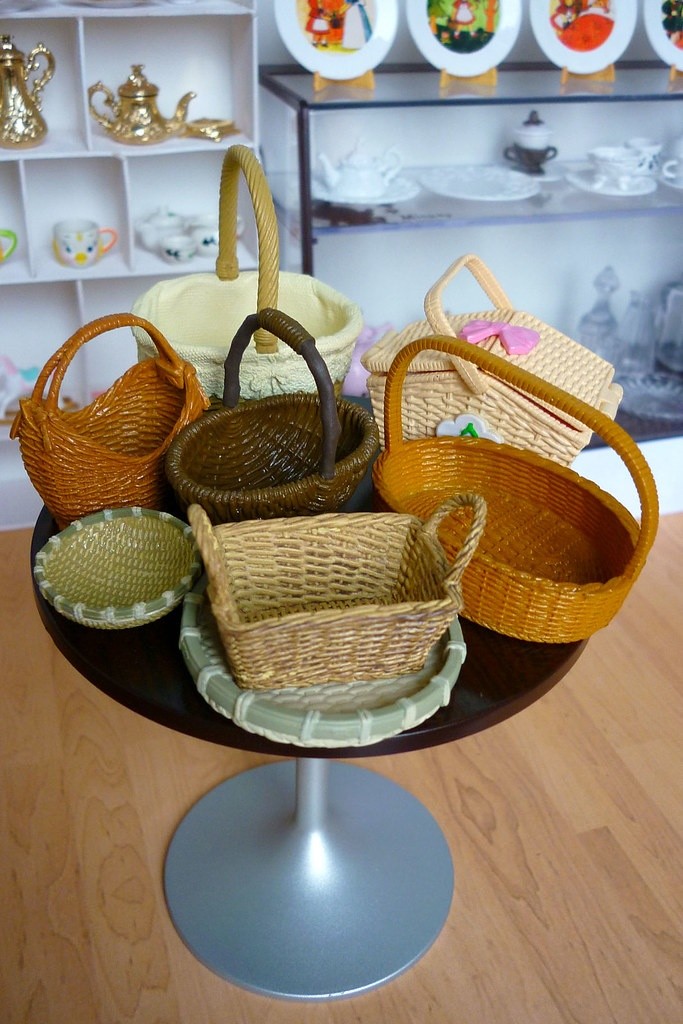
[361,251,623,468]
[178,577,466,750]
[371,335,659,645]
[187,494,487,693]
[164,307,379,526]
[130,144,361,414]
[34,505,202,630]
[9,314,212,531]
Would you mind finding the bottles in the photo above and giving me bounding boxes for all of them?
[574,270,683,377]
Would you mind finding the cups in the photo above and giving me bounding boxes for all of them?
[586,137,683,190]
[53,218,118,269]
[161,215,244,263]
[0,226,17,266]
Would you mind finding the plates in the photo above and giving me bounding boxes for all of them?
[642,0,683,72]
[658,175,683,189]
[272,0,399,80]
[610,372,683,426]
[311,175,419,205]
[420,165,542,202]
[564,169,658,197]
[404,0,522,78]
[526,0,639,76]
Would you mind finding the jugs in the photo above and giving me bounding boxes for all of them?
[317,148,401,200]
[0,34,56,150]
[505,110,558,176]
[87,63,197,147]
[141,200,194,252]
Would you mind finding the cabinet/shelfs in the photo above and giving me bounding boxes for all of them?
[0,0,263,532]
[256,62,683,520]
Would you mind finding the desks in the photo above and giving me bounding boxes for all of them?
[30,394,590,1004]
